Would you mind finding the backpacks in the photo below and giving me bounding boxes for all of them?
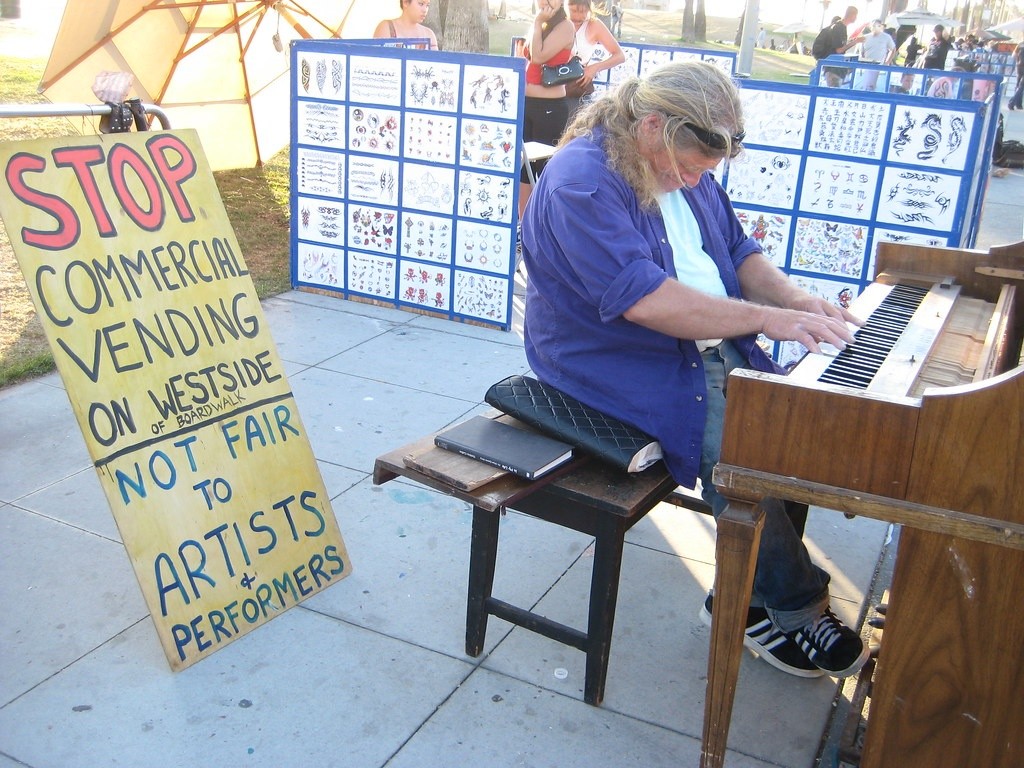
[812,27,834,61]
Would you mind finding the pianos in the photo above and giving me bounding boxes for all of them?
[698,239,1024,768]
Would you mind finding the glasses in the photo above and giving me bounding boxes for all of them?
[660,108,747,150]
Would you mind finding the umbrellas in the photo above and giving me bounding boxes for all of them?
[772,22,813,33]
[37,0,403,170]
[896,6,965,26]
[958,28,1013,41]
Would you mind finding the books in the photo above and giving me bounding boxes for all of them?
[434,415,574,480]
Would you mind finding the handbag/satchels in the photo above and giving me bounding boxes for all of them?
[540,55,584,88]
[566,78,595,98]
[486,374,663,474]
[998,140,1024,168]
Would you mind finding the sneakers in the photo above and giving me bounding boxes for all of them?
[700,584,872,679]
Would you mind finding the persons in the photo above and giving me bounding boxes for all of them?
[895,72,915,93]
[522,61,872,679]
[955,34,987,53]
[904,38,924,67]
[371,0,439,51]
[824,6,896,90]
[924,25,950,69]
[1007,41,1024,110]
[757,28,766,48]
[611,5,624,38]
[517,0,626,245]
[770,39,776,50]
[927,66,965,99]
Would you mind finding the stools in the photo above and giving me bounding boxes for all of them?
[465,443,713,705]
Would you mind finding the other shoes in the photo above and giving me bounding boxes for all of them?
[1008,102,1023,110]
